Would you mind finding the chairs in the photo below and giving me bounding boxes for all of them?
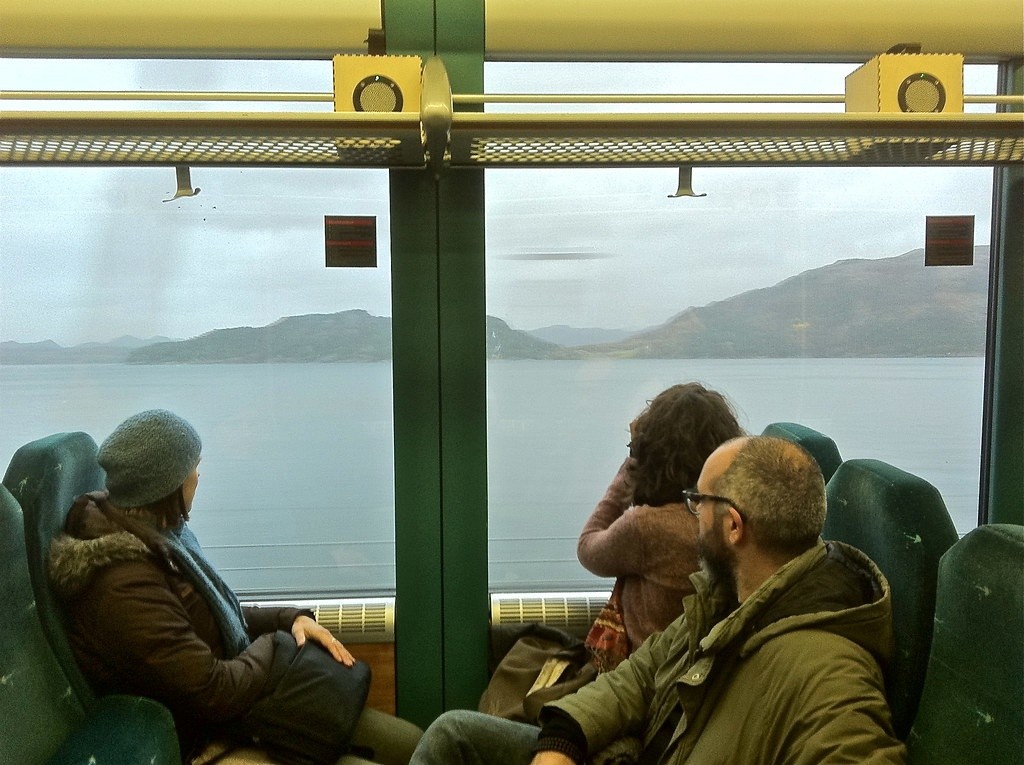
[0,482,184,765]
[0,431,106,711]
[909,525,1024,765]
[758,422,843,575]
[821,460,959,727]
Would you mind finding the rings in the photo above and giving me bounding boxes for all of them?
[332,638,337,642]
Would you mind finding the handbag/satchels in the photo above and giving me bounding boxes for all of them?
[238,628,372,765]
[478,624,600,726]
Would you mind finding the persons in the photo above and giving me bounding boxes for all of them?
[404,434,906,765]
[47,407,424,765]
[498,380,750,728]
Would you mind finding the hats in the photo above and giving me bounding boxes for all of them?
[96,409,202,509]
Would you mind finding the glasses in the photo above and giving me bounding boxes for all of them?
[682,489,747,525]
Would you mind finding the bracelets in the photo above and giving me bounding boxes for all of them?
[533,736,582,763]
